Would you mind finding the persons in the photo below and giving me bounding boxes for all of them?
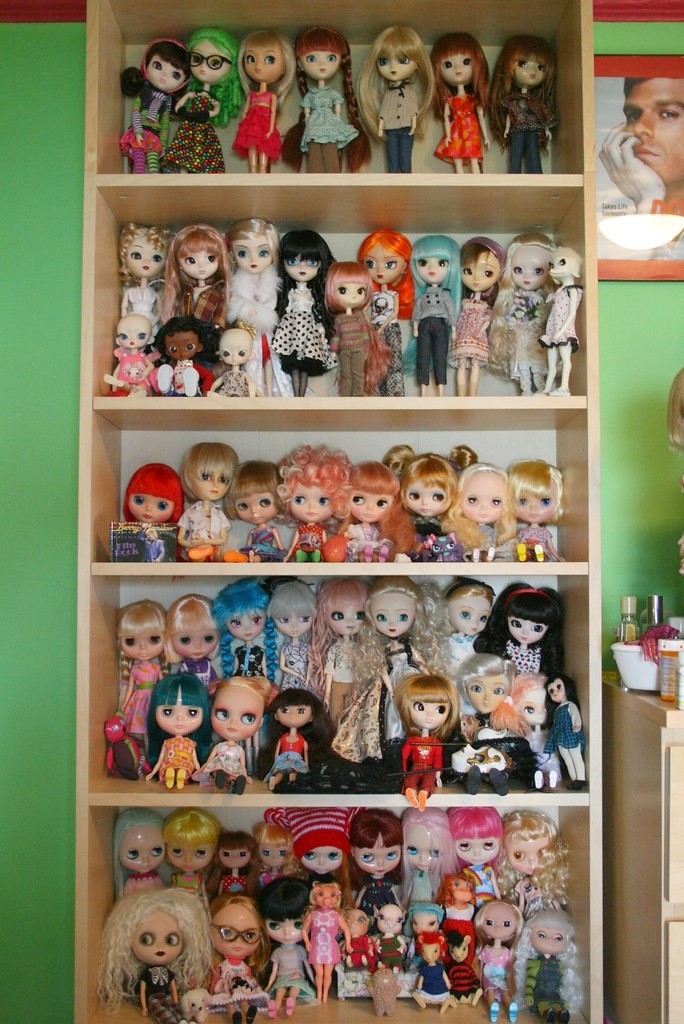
[122,441,248,562]
[343,444,567,563]
[95,804,412,1024]
[443,577,587,796]
[484,233,583,396]
[104,215,281,397]
[118,35,191,173]
[189,575,460,811]
[105,593,220,789]
[137,523,164,563]
[280,23,437,173]
[357,228,508,396]
[598,77,684,259]
[160,27,297,174]
[430,32,559,174]
[400,806,572,920]
[223,442,355,562]
[270,229,393,397]
[473,898,583,1024]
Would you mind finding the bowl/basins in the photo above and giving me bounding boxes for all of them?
[611,641,660,690]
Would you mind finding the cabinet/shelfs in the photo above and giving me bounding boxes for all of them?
[604,676,683,1024]
[65,1,604,1024]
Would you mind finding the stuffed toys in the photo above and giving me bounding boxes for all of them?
[366,969,404,1017]
[180,986,212,1024]
[373,903,407,975]
[408,900,483,1013]
[103,709,153,781]
[340,909,374,968]
[426,531,463,563]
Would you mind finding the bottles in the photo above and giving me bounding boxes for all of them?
[658,638,684,710]
[620,595,638,642]
[640,606,674,638]
